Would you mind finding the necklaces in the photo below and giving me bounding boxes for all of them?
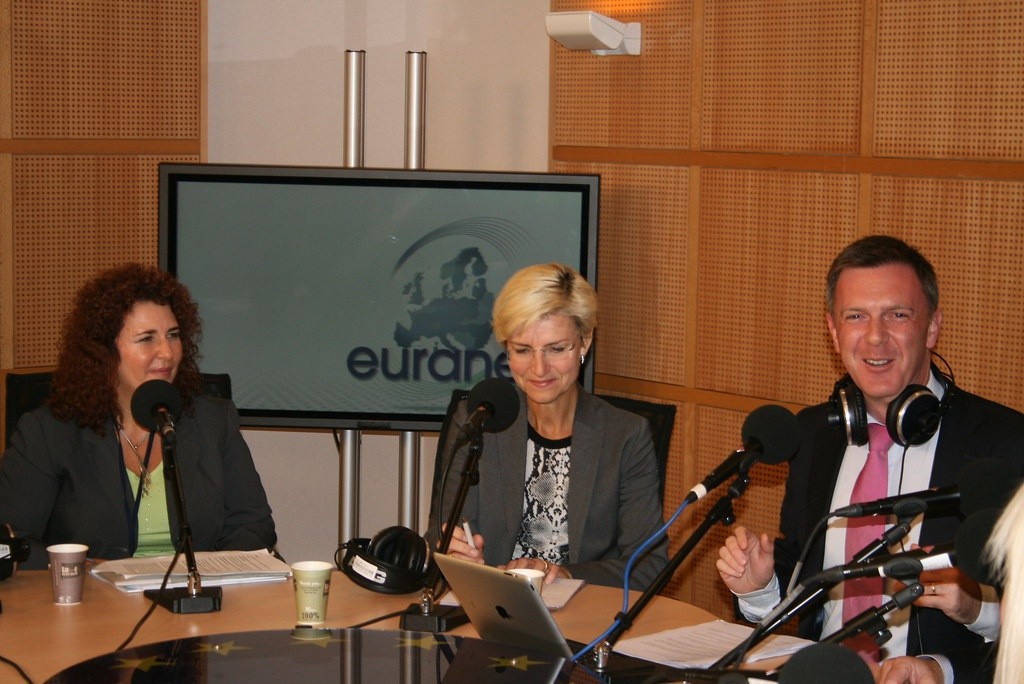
[113,419,153,498]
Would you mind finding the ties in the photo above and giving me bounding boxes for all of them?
[841,422,893,626]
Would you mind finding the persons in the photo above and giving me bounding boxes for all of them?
[422,261,672,596]
[714,235,1024,684]
[0,260,278,571]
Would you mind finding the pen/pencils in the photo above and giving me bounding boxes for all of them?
[461,516,475,547]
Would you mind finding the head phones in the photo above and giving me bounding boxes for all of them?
[824,349,956,446]
[345,525,430,594]
[0,524,30,581]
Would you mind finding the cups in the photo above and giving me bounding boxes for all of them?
[47,543,89,604]
[290,560,334,624]
[507,569,545,596]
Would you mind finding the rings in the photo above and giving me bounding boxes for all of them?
[931,582,936,592]
[446,551,455,558]
[541,560,549,572]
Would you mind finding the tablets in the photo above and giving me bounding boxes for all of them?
[433,552,576,665]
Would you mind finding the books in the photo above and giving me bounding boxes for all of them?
[88,547,294,593]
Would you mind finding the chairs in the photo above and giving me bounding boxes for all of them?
[429,388,676,508]
[0,366,233,544]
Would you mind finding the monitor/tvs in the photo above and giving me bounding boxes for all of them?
[156,162,602,432]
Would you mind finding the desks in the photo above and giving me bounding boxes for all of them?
[0,570,791,684]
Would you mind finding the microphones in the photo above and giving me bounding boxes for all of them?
[684,404,802,504]
[816,542,959,583]
[834,483,961,518]
[779,643,877,684]
[453,377,519,446]
[132,378,181,442]
